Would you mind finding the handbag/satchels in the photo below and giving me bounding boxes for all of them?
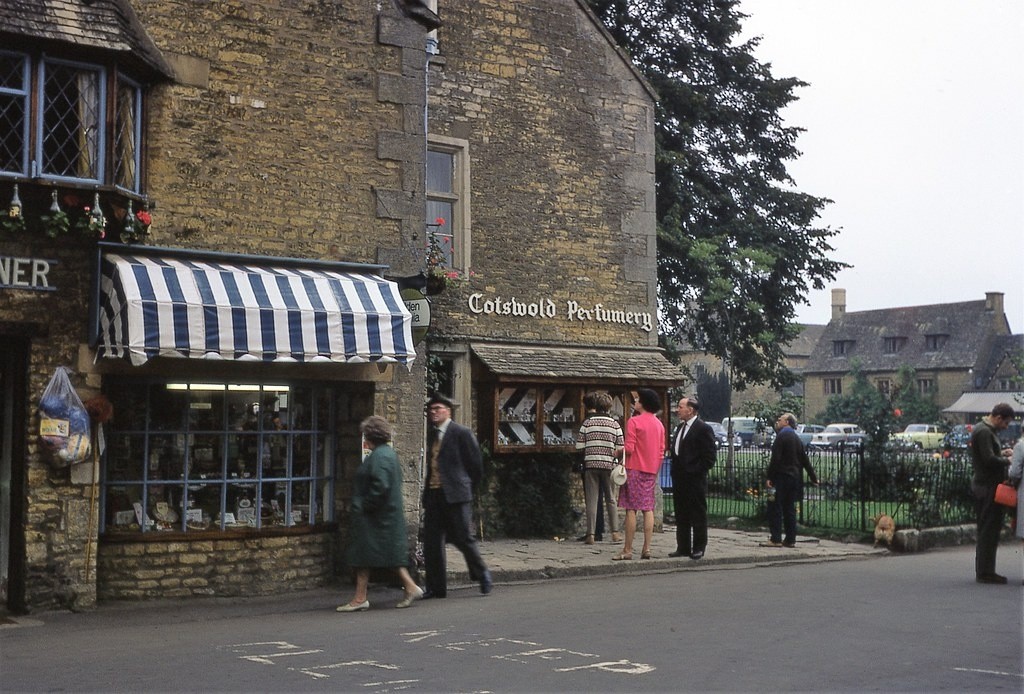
[610,448,627,486]
[993,480,1017,508]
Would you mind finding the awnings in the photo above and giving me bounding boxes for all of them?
[942,390,1024,412]
[470,342,688,381]
[92,251,417,373]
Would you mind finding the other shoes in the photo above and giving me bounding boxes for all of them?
[613,534,622,541]
[577,533,603,541]
[584,537,594,544]
[781,540,795,548]
[759,541,782,547]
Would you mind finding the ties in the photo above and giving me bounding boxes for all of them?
[679,423,687,451]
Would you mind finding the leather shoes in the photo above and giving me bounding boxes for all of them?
[668,548,704,559]
[481,571,493,593]
[423,587,446,598]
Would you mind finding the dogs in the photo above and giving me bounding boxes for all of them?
[869,513,895,549]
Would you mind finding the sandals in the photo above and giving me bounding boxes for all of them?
[641,550,650,559]
[612,553,632,560]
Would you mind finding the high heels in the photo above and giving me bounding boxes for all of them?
[395,587,424,608]
[336,600,369,612]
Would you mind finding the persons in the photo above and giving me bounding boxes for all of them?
[757,414,820,547]
[575,392,625,543]
[1006,433,1024,538]
[668,398,717,560]
[968,402,1017,584]
[417,394,493,600]
[576,393,606,541]
[613,388,666,560]
[335,415,424,612]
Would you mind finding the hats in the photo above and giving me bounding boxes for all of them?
[425,392,461,409]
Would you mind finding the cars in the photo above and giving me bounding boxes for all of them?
[943,424,974,453]
[723,416,768,447]
[810,422,873,452]
[704,421,742,451]
[888,424,947,452]
[771,423,826,451]
